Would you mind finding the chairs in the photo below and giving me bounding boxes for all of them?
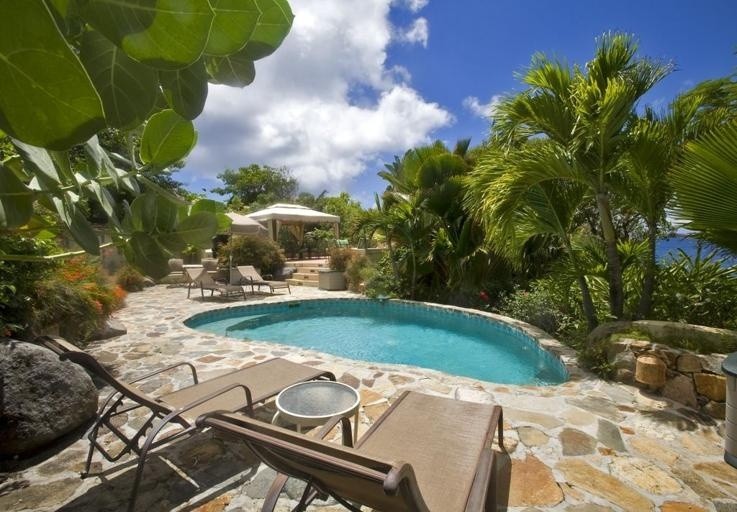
[283,235,322,260]
[194,385,513,512]
[33,332,338,511]
[236,265,293,297]
[181,262,247,302]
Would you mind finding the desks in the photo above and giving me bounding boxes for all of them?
[270,377,362,452]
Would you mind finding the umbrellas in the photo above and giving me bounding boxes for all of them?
[223,212,266,268]
